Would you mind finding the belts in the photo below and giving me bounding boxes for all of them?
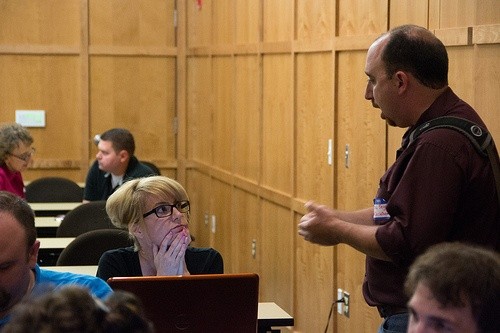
[376,303,410,318]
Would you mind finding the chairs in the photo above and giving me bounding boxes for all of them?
[25,176,83,202]
[56,228,134,266]
[56,201,114,238]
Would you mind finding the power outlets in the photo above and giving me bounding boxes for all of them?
[343,291,350,319]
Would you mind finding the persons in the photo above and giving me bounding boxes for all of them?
[0,286,156,333]
[404,243,500,333]
[94,177,224,279]
[0,192,120,333]
[295,24,500,333]
[82,128,156,204]
[0,123,36,202]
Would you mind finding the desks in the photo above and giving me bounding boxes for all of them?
[257,302,294,333]
[28,202,98,276]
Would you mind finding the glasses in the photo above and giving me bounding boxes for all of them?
[6,147,36,161]
[135,201,190,224]
